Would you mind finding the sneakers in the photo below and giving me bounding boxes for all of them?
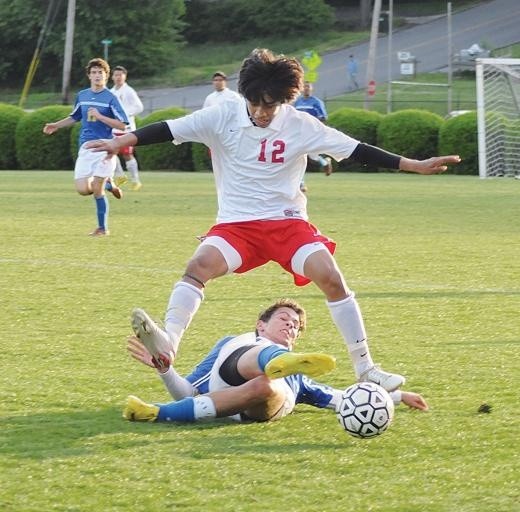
[324,156,332,175]
[131,308,176,365]
[130,182,141,191]
[300,179,307,192]
[357,364,405,392]
[105,178,122,199]
[123,395,161,422]
[263,352,336,379]
[115,176,128,188]
[89,228,110,237]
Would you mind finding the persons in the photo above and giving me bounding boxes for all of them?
[292,81,332,191]
[82,48,462,393]
[43,58,130,238]
[122,297,429,422]
[201,71,246,110]
[108,65,145,191]
[346,55,361,92]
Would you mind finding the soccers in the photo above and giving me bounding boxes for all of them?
[337,382,394,439]
